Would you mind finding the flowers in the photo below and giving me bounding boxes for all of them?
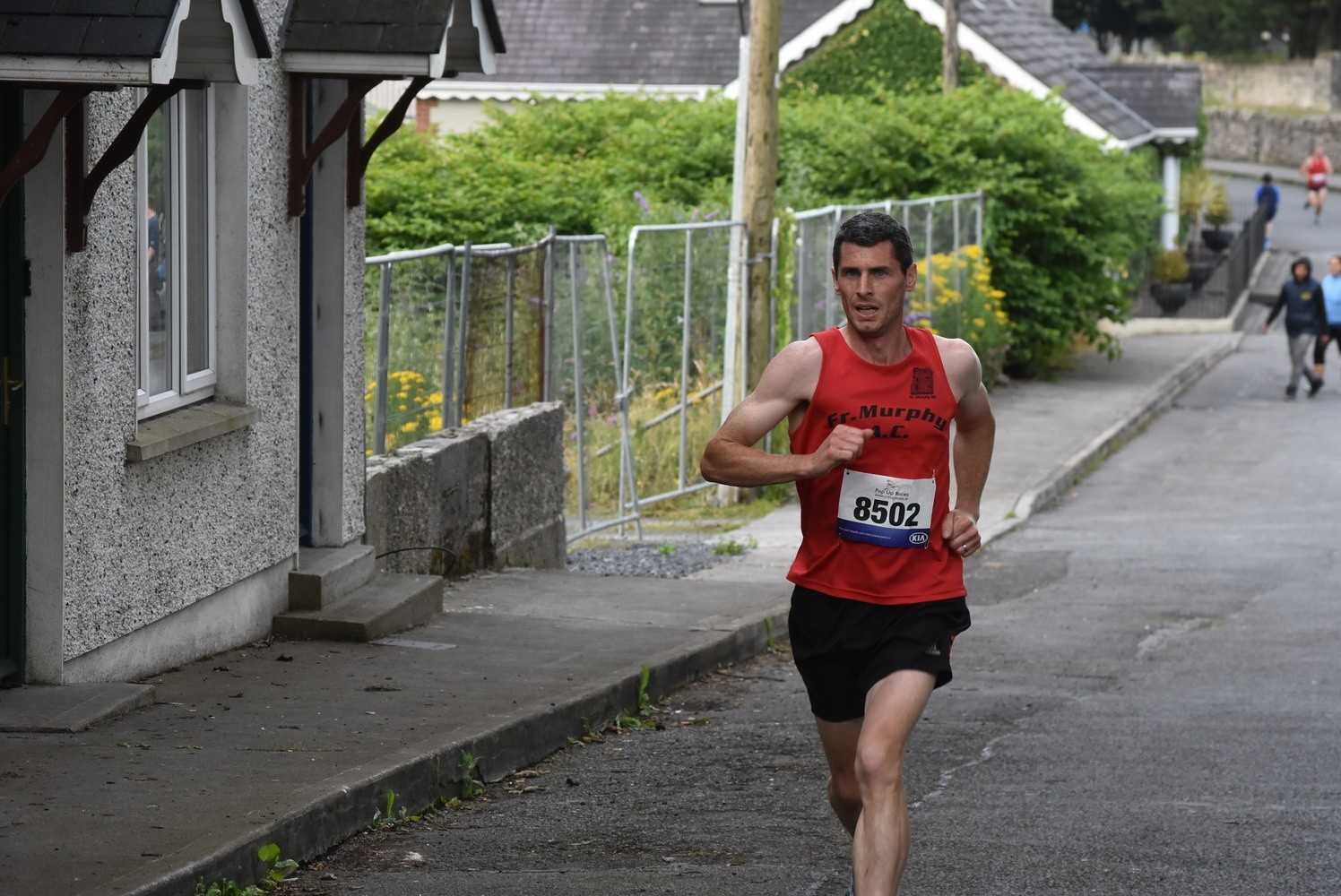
[1204,182,1234,231]
[1151,248,1189,286]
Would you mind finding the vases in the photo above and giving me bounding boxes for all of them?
[1200,229,1234,252]
[1150,283,1193,317]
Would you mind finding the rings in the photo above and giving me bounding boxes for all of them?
[962,544,968,553]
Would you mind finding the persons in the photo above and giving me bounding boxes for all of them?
[1300,144,1333,226]
[1261,256,1329,399]
[1303,256,1341,393]
[701,211,995,896]
[1254,172,1282,253]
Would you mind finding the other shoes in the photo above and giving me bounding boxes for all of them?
[1286,385,1297,396]
[1308,380,1323,397]
